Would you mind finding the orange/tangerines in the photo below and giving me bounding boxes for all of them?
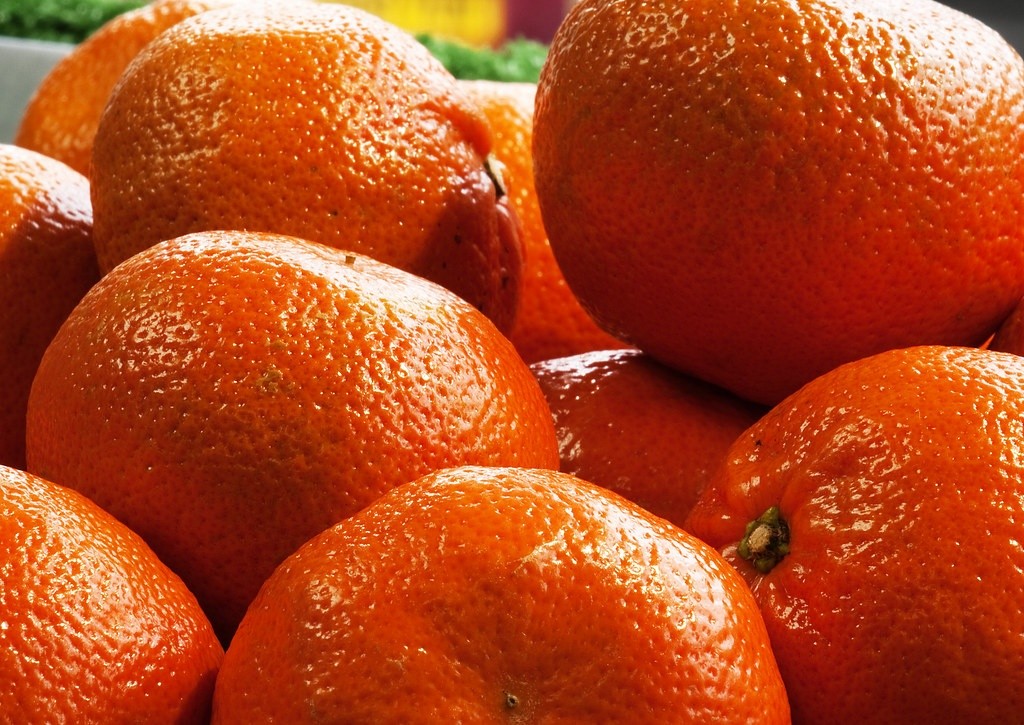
[1,0,1023,725]
